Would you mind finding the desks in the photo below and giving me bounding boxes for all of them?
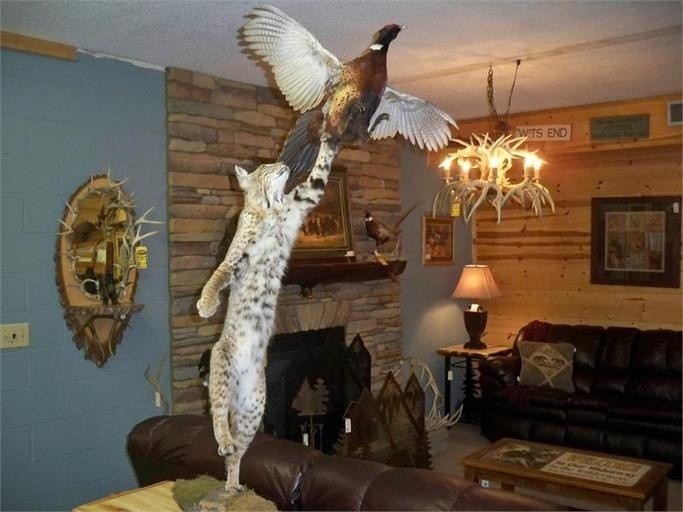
[70,477,187,511]
[434,342,508,432]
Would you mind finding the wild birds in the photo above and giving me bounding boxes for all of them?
[363,198,426,253]
[242,4,459,194]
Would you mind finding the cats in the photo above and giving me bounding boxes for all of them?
[196,134,338,494]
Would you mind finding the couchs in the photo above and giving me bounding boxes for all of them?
[478,319,682,484]
[123,411,575,511]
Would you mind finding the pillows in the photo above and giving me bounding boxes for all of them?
[515,337,581,394]
[571,366,602,396]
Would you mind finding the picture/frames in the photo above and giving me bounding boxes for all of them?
[419,213,456,268]
[590,194,683,290]
[250,152,354,260]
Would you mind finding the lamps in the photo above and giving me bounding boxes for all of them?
[451,262,505,352]
[429,57,557,224]
